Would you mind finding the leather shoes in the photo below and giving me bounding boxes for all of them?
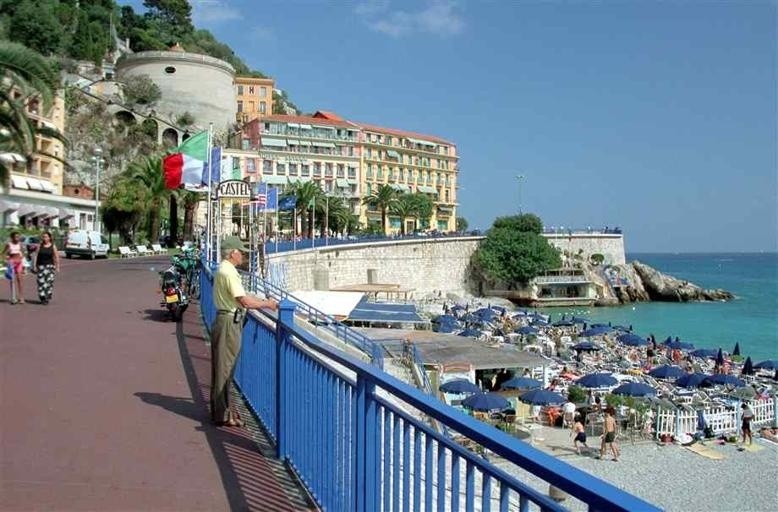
[215,418,246,427]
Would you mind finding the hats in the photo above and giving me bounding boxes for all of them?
[220,236,253,252]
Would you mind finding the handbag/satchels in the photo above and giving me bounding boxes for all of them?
[4,264,15,280]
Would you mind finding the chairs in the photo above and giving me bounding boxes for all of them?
[116,242,168,259]
[479,311,777,432]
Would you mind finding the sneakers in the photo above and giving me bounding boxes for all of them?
[611,458,618,462]
[11,298,25,305]
[41,296,52,304]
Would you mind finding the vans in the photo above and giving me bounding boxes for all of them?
[65,229,111,259]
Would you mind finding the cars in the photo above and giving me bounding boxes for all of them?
[19,236,41,246]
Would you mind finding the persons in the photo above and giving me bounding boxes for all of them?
[0,231,60,305]
[567,415,591,452]
[738,402,756,445]
[207,236,282,428]
[595,406,622,463]
[759,426,778,442]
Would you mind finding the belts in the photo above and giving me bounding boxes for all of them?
[216,310,235,317]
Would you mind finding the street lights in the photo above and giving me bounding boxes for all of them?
[93,148,103,232]
[512,175,528,214]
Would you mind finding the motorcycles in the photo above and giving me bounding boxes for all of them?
[149,246,203,322]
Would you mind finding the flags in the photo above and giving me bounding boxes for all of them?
[240,181,299,214]
[160,130,220,189]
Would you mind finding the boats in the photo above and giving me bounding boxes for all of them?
[529,267,599,306]
